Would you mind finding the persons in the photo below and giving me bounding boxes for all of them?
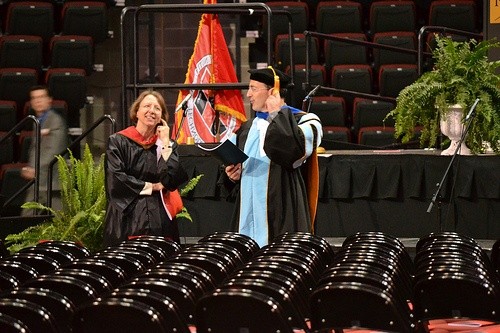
[225,66,323,249]
[104,90,184,245]
[20,87,68,215]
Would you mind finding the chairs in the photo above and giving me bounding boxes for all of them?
[0,232,500,333]
[257,0,477,146]
[0,2,107,208]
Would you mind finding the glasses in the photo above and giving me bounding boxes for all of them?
[248,85,269,92]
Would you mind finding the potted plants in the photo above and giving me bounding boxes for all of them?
[384,32,500,156]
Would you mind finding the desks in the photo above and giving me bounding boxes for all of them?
[175,144,500,237]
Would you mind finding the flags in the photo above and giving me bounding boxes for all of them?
[171,0,247,146]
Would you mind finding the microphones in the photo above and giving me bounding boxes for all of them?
[304,85,320,101]
[466,99,479,120]
[175,94,191,112]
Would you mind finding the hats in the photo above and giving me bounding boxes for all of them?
[247,66,293,93]
